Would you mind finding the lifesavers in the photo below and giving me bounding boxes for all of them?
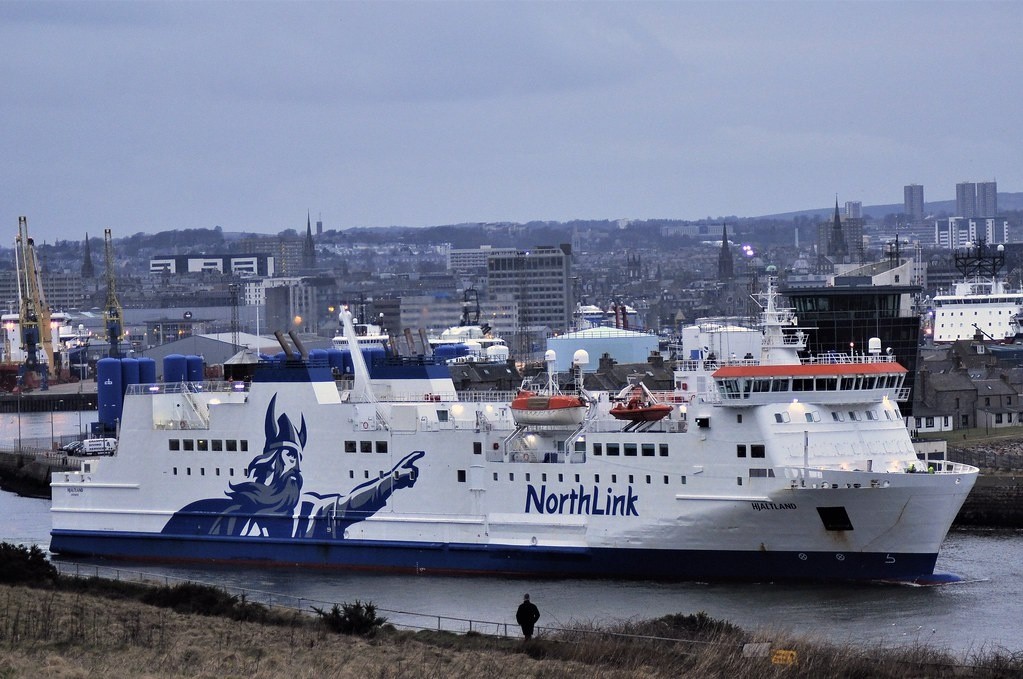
[363,422,368,429]
[180,421,185,429]
[524,454,529,461]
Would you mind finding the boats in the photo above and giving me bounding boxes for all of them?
[49,276,981,584]
[609,403,674,421]
[507,391,589,426]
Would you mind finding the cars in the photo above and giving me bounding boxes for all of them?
[69,442,83,455]
[94,387,98,392]
[67,442,79,452]
[62,441,80,450]
[74,445,84,456]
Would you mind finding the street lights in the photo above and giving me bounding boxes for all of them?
[45,400,64,450]
[77,353,83,392]
[73,403,92,441]
[16,376,23,452]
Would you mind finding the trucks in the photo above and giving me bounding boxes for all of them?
[82,438,117,456]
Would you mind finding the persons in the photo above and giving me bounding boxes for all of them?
[907,464,916,473]
[244,375,250,391]
[424,393,441,402]
[731,352,736,359]
[536,390,543,397]
[207,379,217,391]
[928,464,934,474]
[229,375,233,390]
[187,381,193,391]
[514,388,520,398]
